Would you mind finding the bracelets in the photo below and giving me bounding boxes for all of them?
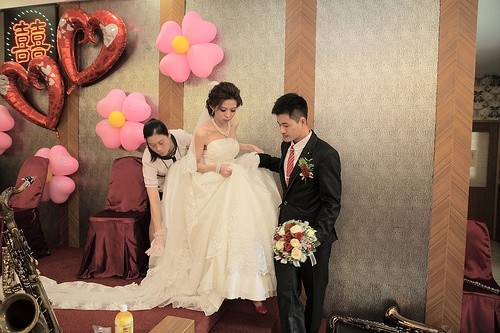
[215,163,222,173]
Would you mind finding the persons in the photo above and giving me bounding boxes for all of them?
[142,118,192,234]
[184,81,268,315]
[255,93,342,333]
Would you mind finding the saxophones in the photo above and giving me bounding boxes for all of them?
[0,176,63,333]
[329,305,441,333]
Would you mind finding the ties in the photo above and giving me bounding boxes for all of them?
[287,146,294,185]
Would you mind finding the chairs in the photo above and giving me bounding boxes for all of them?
[76,156,151,280]
[460,218,500,333]
[0,156,51,276]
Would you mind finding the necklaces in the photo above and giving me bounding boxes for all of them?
[211,119,231,137]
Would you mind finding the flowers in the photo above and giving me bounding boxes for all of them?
[298,157,314,185]
[271,219,321,267]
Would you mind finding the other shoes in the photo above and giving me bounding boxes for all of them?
[253,302,267,314]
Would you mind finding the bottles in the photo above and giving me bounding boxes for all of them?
[114,303,133,333]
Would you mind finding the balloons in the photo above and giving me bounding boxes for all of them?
[0,9,224,204]
